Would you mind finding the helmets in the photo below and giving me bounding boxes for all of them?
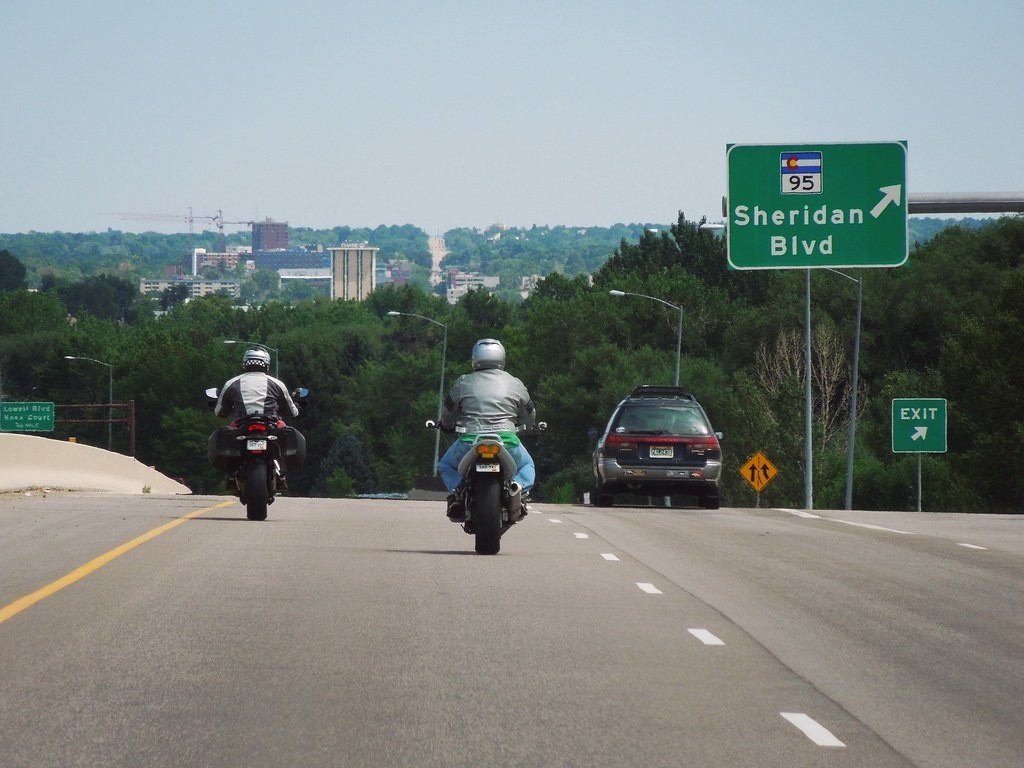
[242,346,270,375]
[471,338,506,371]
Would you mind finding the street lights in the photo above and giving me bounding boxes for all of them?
[609,290,683,507]
[65,355,113,451]
[388,310,448,479]
[225,340,279,385]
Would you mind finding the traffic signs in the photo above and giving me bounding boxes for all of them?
[725,141,910,270]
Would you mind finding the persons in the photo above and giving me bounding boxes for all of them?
[214,346,300,490]
[437,339,536,519]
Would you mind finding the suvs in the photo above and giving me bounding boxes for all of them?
[593,385,724,509]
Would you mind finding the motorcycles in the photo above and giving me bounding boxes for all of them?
[205,387,308,521]
[426,419,549,555]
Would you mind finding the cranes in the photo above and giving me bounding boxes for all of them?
[93,207,252,277]
